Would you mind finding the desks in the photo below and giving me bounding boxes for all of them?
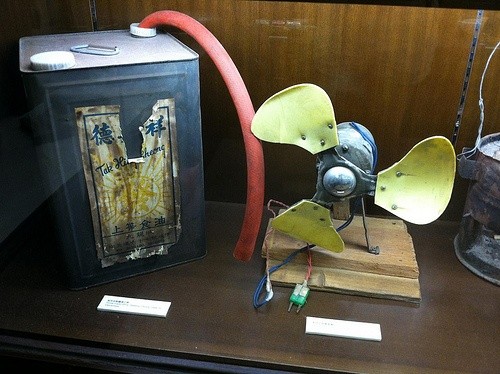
[0,200,499,374]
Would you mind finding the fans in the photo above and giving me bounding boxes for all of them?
[249,82,457,256]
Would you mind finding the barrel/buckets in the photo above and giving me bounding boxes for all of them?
[18,23,208,291]
